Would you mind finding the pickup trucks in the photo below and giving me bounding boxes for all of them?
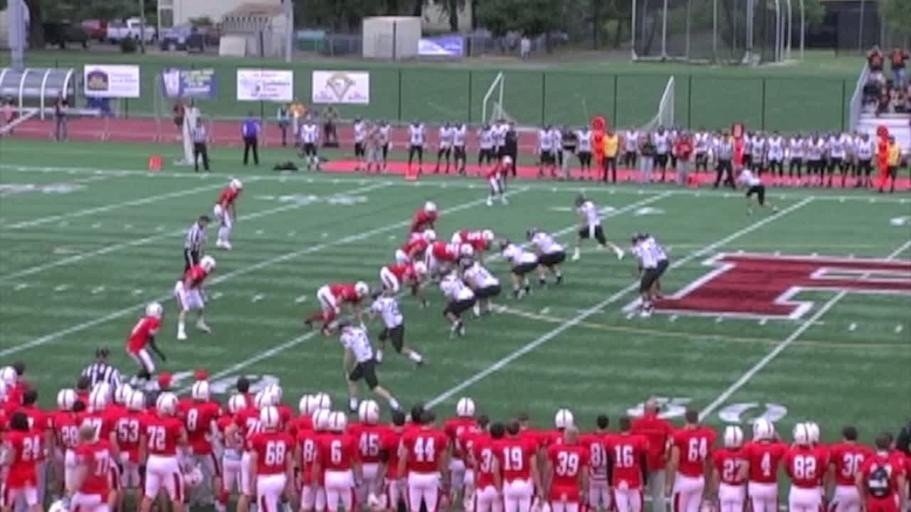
[106,17,157,47]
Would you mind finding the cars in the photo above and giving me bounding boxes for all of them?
[160,22,216,51]
[82,18,107,43]
[43,19,91,50]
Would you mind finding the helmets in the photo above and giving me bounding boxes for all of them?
[423,229,434,242]
[146,302,164,318]
[457,397,474,417]
[499,240,508,248]
[155,392,178,415]
[482,230,493,242]
[555,407,573,428]
[360,400,379,424]
[228,384,282,427]
[230,178,242,193]
[437,244,474,276]
[202,255,216,273]
[58,379,145,413]
[300,393,346,433]
[527,229,537,236]
[192,382,212,401]
[724,419,819,449]
[370,285,383,296]
[338,317,350,325]
[424,201,435,213]
[354,282,369,299]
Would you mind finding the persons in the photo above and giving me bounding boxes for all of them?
[571,194,668,317]
[522,35,529,61]
[127,179,242,375]
[354,114,519,176]
[864,43,911,115]
[1,94,118,141]
[443,397,911,512]
[0,342,446,512]
[537,123,619,184]
[305,199,566,412]
[487,155,513,205]
[621,124,902,216]
[173,97,210,171]
[242,103,339,171]
